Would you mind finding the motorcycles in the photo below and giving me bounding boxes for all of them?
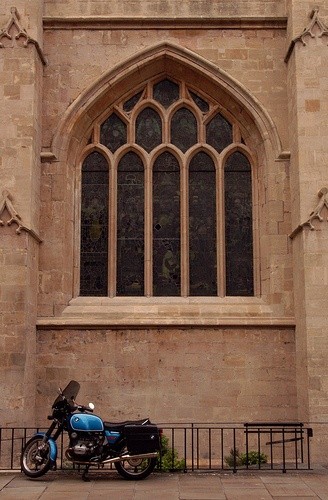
[20,380,167,481]
